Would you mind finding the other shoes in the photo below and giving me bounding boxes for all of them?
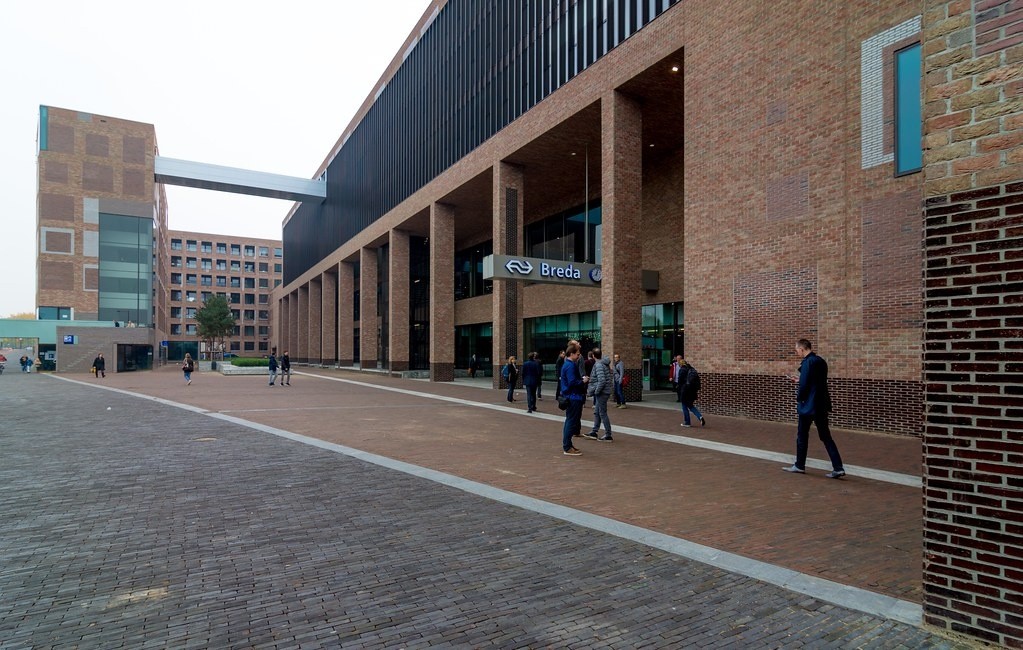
[538,396,542,400]
[285,383,290,386]
[680,423,690,427]
[700,417,706,426]
[103,374,106,377]
[532,407,537,411]
[597,434,613,442]
[280,383,283,386]
[187,380,192,385]
[616,404,620,406]
[618,404,627,409]
[269,382,275,387]
[527,411,532,413]
[583,432,598,439]
[563,445,583,455]
[572,433,585,437]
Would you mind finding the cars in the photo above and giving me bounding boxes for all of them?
[224,353,240,358]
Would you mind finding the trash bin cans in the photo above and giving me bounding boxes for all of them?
[211,362,217,370]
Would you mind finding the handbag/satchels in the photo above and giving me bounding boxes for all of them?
[558,396,570,410]
[621,374,630,386]
[182,359,189,370]
[91,366,96,373]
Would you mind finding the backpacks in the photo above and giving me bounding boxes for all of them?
[683,367,698,391]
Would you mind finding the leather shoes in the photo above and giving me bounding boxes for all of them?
[781,465,806,474]
[825,470,845,478]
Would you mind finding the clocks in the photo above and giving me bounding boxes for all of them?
[590,267,602,282]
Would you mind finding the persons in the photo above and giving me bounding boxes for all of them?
[183,353,194,384]
[469,353,479,378]
[556,341,588,437]
[268,352,279,387]
[92,353,105,378]
[783,338,845,478]
[678,359,705,427]
[522,354,544,413]
[507,356,518,402]
[0,353,7,369]
[582,348,613,442]
[561,344,590,455]
[670,355,682,392]
[20,356,42,372]
[127,321,133,328]
[113,319,119,327]
[613,354,626,409]
[280,351,290,385]
